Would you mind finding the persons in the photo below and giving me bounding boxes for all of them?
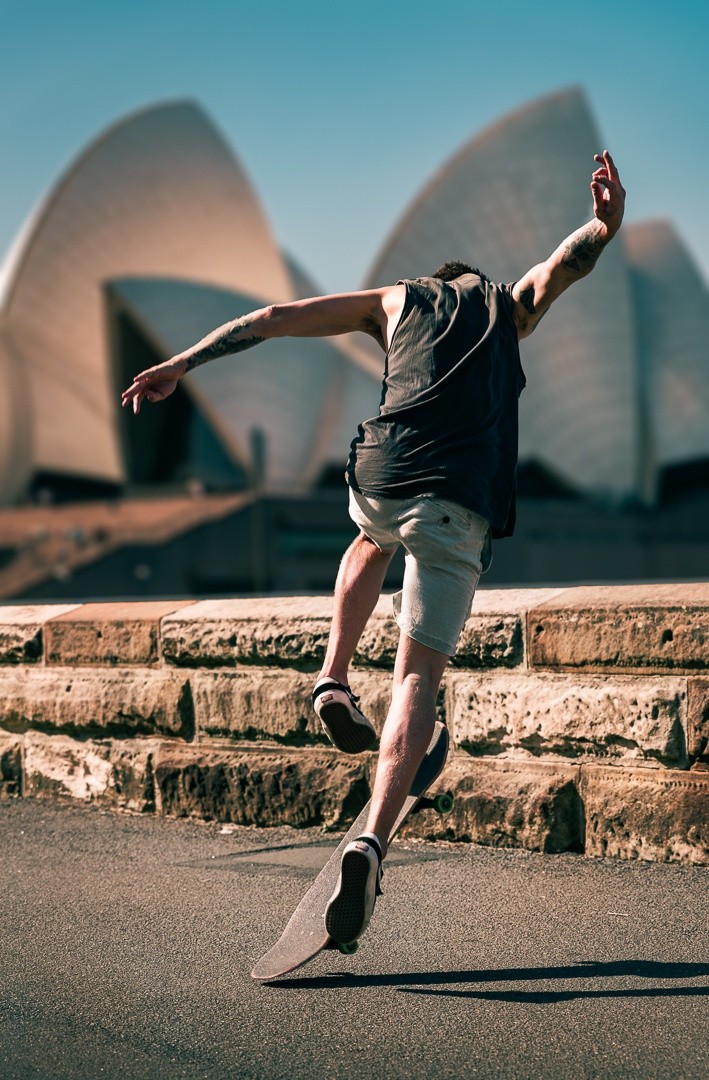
[119,150,628,945]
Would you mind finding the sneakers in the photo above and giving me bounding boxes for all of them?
[312,675,378,755]
[323,833,384,944]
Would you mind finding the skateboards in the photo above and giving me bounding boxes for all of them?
[250,720,454,981]
